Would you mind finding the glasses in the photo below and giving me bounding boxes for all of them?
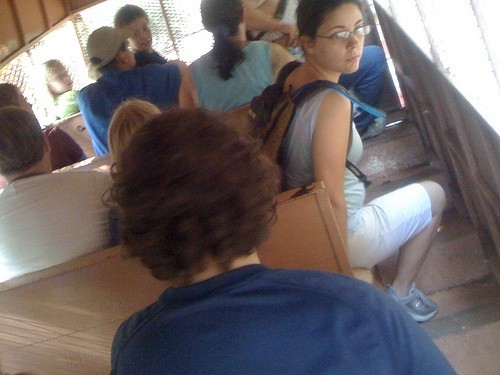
[312,24,372,42]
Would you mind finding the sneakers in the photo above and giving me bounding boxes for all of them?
[384,282,439,324]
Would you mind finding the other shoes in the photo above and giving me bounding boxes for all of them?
[359,114,388,140]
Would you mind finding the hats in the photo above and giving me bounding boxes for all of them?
[85,26,136,68]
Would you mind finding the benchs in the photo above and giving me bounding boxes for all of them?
[0,112,351,375]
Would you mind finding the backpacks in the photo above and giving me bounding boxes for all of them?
[249,60,354,164]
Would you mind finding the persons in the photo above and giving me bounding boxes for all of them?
[0,0,387,282]
[273,1,447,322]
[100,107,456,375]
